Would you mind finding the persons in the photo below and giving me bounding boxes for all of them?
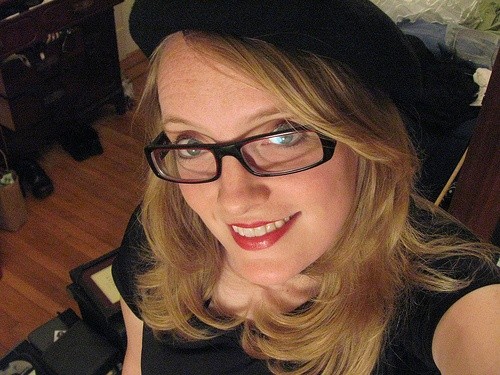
[111,0,500,375]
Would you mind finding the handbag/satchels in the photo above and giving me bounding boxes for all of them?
[0,150,28,233]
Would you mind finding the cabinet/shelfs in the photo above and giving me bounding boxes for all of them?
[0,1,126,165]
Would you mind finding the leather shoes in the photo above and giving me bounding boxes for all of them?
[16,154,54,199]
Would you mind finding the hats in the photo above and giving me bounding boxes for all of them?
[128,0,417,94]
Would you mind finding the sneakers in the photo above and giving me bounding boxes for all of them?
[59,123,103,162]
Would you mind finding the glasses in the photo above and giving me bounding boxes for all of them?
[143,121,339,184]
